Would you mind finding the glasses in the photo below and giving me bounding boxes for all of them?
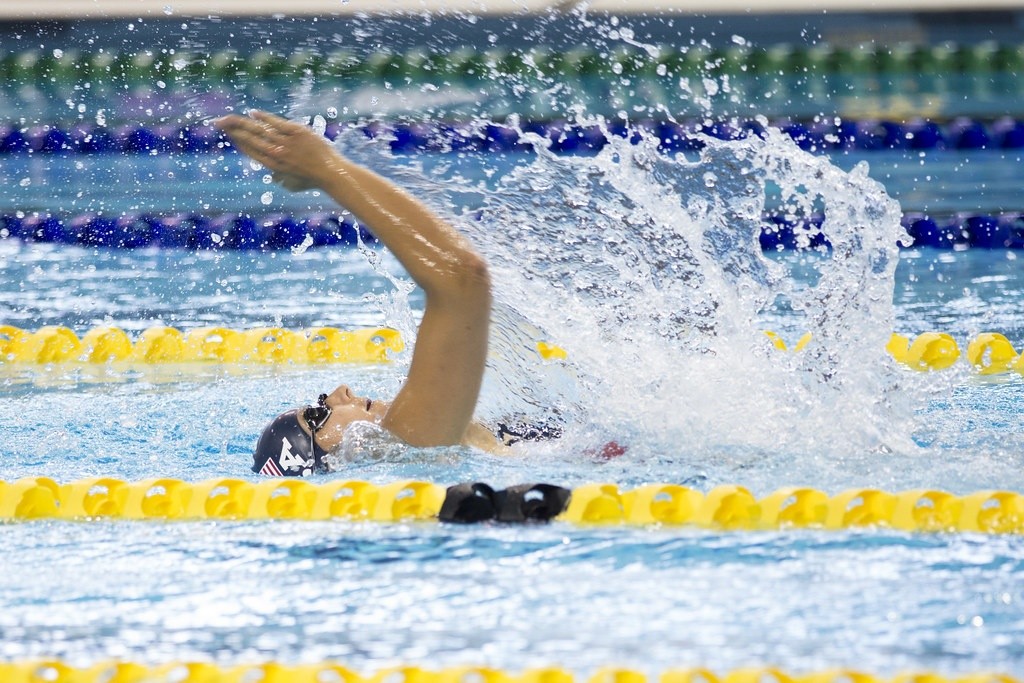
[301,394,333,470]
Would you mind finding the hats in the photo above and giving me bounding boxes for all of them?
[251,410,328,476]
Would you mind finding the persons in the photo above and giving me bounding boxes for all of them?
[216,110,506,476]
